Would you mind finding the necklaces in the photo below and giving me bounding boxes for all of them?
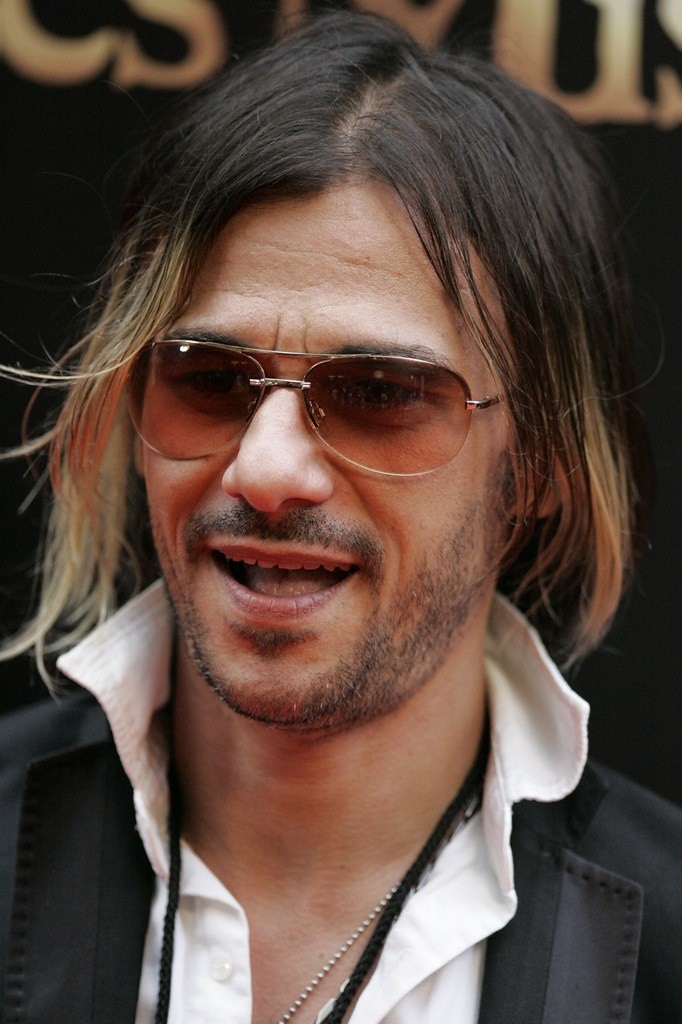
[153,741,489,1024]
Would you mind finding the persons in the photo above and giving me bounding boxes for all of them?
[0,11,681,1024]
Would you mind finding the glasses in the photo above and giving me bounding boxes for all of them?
[122,338,527,478]
